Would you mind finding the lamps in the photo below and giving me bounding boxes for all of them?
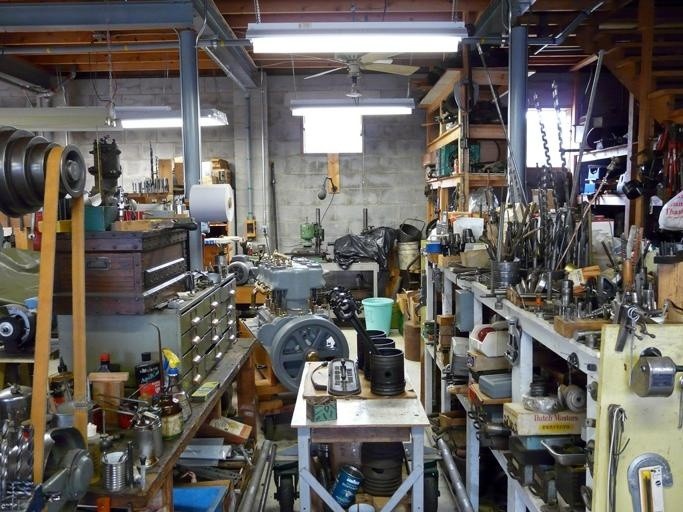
[290,54,416,117]
[346,76,362,97]
[1,52,116,131]
[121,70,228,129]
[246,1,469,54]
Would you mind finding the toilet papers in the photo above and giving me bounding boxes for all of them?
[190,184,234,224]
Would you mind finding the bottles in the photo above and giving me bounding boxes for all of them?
[354,274,362,287]
[132,350,183,460]
[96,353,111,372]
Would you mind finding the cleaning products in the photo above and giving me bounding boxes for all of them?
[161,349,193,423]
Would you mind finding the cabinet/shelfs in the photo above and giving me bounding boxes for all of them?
[570,42,638,235]
[0,337,259,512]
[423,246,683,512]
[56,272,236,400]
[417,67,509,221]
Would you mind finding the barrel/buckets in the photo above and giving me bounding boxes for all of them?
[398,241,420,271]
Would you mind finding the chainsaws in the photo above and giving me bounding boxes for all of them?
[19,425,96,512]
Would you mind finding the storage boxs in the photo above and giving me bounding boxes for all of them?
[502,403,586,435]
[172,479,236,512]
[53,230,192,316]
[203,237,234,265]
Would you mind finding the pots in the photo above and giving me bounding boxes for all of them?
[0,385,33,424]
[50,399,96,428]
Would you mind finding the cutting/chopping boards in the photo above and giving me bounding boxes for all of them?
[300,356,415,401]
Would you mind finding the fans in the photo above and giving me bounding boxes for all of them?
[303,0,420,80]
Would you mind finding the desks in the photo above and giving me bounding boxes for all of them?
[290,361,430,512]
[292,257,379,299]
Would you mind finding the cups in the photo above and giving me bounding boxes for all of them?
[439,227,472,247]
[354,326,406,397]
[134,211,143,219]
[101,451,130,494]
[123,210,132,220]
[488,256,521,292]
